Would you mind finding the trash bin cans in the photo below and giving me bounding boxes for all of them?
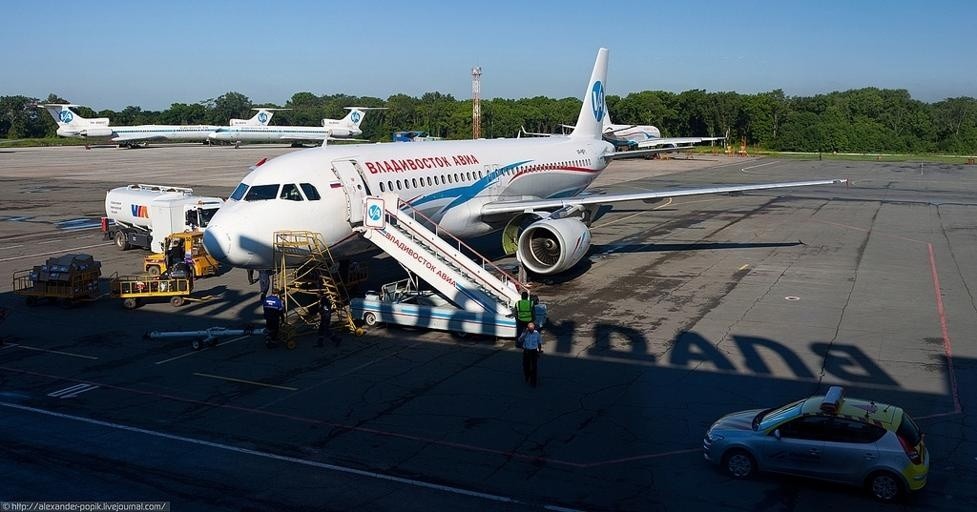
[968,159,974,165]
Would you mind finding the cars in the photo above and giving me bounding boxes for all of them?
[700,387,932,504]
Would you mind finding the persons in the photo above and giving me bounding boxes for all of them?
[259,270,276,299]
[246,268,254,285]
[263,288,284,331]
[517,323,543,387]
[514,291,535,348]
[534,295,548,333]
[313,297,343,347]
[169,240,183,264]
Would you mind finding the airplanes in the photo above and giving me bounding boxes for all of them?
[518,123,729,160]
[41,105,389,148]
[201,49,849,338]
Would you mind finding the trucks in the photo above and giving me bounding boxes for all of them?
[100,183,224,253]
